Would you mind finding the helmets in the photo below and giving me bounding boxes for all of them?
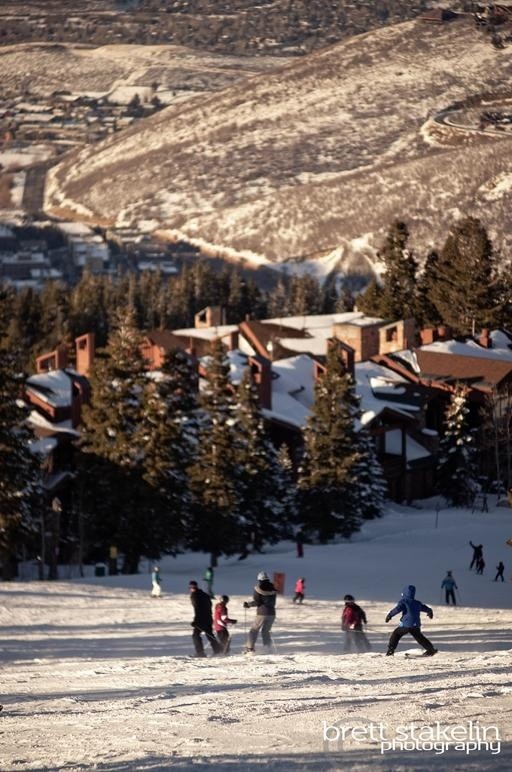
[257,571,269,580]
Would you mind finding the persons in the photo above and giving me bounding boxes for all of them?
[149,565,163,598]
[204,565,215,598]
[339,594,372,653]
[439,569,458,604]
[385,583,438,656]
[292,576,306,604]
[188,580,222,658]
[468,540,483,568]
[241,570,278,654]
[494,560,505,582]
[477,557,486,574]
[213,594,237,654]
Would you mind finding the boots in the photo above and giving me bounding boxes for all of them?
[191,652,204,657]
[424,648,439,656]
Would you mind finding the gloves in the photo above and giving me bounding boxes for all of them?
[244,601,248,608]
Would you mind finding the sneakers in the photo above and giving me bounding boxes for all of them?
[244,649,255,655]
